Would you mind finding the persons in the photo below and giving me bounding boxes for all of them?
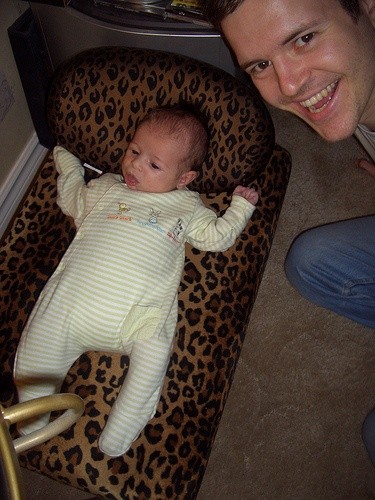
[201,0,375,467]
[14,103,258,458]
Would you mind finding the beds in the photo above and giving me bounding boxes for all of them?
[1,42,294,499]
